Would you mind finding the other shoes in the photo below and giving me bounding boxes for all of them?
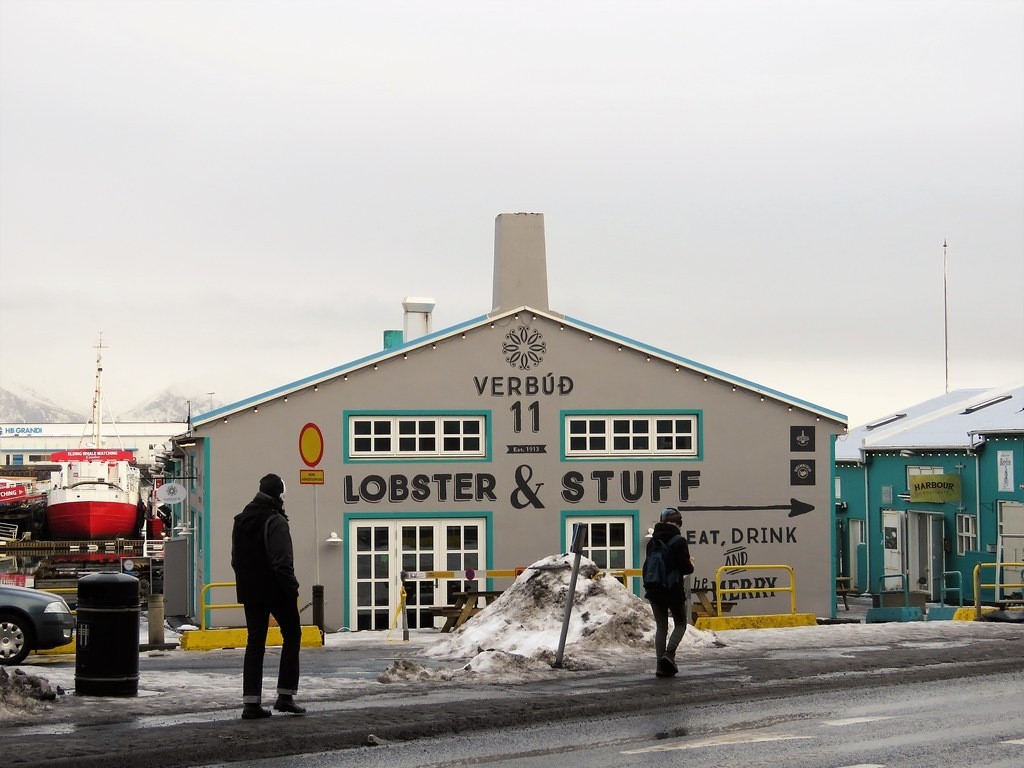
[273,694,306,713]
[241,703,272,719]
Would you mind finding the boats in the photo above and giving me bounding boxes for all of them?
[47,330,140,539]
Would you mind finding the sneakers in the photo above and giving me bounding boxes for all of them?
[661,652,679,673]
[655,665,666,677]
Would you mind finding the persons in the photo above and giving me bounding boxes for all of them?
[642,508,694,678]
[230,473,304,721]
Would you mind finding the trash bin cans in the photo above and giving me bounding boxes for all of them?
[74,570,140,698]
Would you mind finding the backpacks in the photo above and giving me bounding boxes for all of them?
[642,534,687,600]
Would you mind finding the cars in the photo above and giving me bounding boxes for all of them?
[0,584,74,665]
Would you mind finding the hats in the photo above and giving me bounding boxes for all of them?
[259,473,284,499]
